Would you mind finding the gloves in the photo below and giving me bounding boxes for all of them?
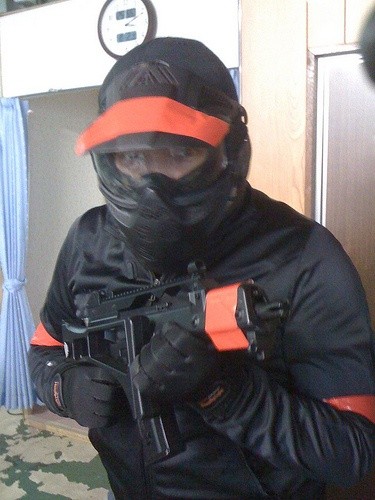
[130,321,258,422]
[44,364,126,430]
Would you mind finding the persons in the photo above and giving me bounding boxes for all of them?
[28,37,375,500]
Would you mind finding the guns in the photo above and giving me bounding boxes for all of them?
[57,259,293,462]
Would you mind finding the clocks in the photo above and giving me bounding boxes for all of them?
[97,0,158,60]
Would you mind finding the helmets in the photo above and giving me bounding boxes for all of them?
[73,36,251,275]
